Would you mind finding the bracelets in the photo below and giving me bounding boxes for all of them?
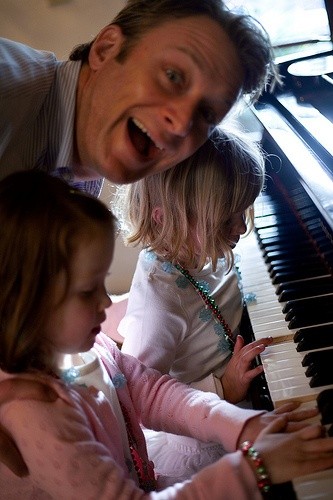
[240,442,272,492]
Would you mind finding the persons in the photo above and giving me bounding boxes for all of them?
[115,124,272,485]
[1,169,332,499]
[0,1,273,481]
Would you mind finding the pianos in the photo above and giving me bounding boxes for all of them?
[210,40,333,499]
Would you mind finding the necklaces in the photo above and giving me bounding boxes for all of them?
[160,252,245,360]
[115,394,153,489]
